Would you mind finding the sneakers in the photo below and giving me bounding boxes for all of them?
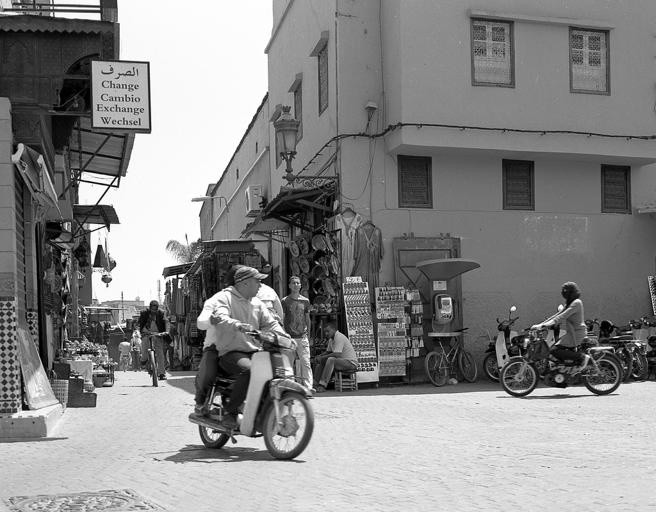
[221,407,236,429]
[580,353,591,372]
[158,373,169,381]
[302,381,328,399]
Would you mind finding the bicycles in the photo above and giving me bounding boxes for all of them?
[139,331,166,387]
[121,353,129,373]
[422,326,478,387]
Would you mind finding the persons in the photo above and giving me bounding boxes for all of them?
[280,275,317,398]
[193,264,240,417]
[312,321,358,393]
[213,266,297,429]
[530,281,591,375]
[118,299,178,381]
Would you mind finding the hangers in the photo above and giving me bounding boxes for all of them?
[361,218,376,229]
[341,203,357,216]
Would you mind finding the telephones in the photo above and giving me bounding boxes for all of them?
[433,294,453,324]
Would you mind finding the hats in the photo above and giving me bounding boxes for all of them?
[233,266,268,283]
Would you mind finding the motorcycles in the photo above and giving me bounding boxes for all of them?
[187,325,314,462]
[482,304,655,399]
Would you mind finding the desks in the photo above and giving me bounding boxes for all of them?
[92,362,118,385]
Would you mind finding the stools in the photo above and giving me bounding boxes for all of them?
[335,371,358,392]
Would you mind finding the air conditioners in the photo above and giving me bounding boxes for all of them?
[244,185,264,218]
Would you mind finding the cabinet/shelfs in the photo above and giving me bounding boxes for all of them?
[310,312,340,388]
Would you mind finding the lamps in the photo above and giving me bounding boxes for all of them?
[273,106,340,183]
[192,196,228,213]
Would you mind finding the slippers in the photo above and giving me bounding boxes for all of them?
[194,403,207,417]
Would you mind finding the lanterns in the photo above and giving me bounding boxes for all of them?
[101,275,112,288]
